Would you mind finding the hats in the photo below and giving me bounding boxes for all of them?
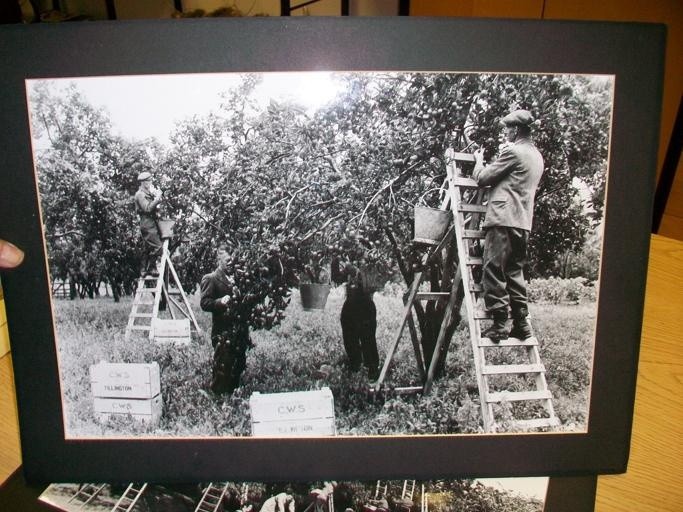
[138,172,151,181]
[502,110,534,127]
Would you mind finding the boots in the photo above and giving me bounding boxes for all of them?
[139,254,159,277]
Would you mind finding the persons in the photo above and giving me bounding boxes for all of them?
[470,109,546,344]
[0,241,26,273]
[199,245,256,394]
[366,491,388,511]
[329,238,380,384]
[391,491,413,511]
[134,170,164,280]
[234,500,254,512]
[258,485,295,512]
[309,480,336,500]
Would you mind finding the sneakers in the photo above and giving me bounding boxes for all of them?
[481,324,532,340]
[350,361,379,383]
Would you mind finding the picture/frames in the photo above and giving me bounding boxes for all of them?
[1,462,598,512]
[1,17,668,484]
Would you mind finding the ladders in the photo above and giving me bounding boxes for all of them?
[121,222,204,352]
[369,150,569,429]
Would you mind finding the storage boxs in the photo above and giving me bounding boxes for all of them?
[88,318,336,437]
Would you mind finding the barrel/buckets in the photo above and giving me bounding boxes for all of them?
[414,187,452,244]
[158,218,176,239]
[301,263,329,313]
[360,263,385,295]
